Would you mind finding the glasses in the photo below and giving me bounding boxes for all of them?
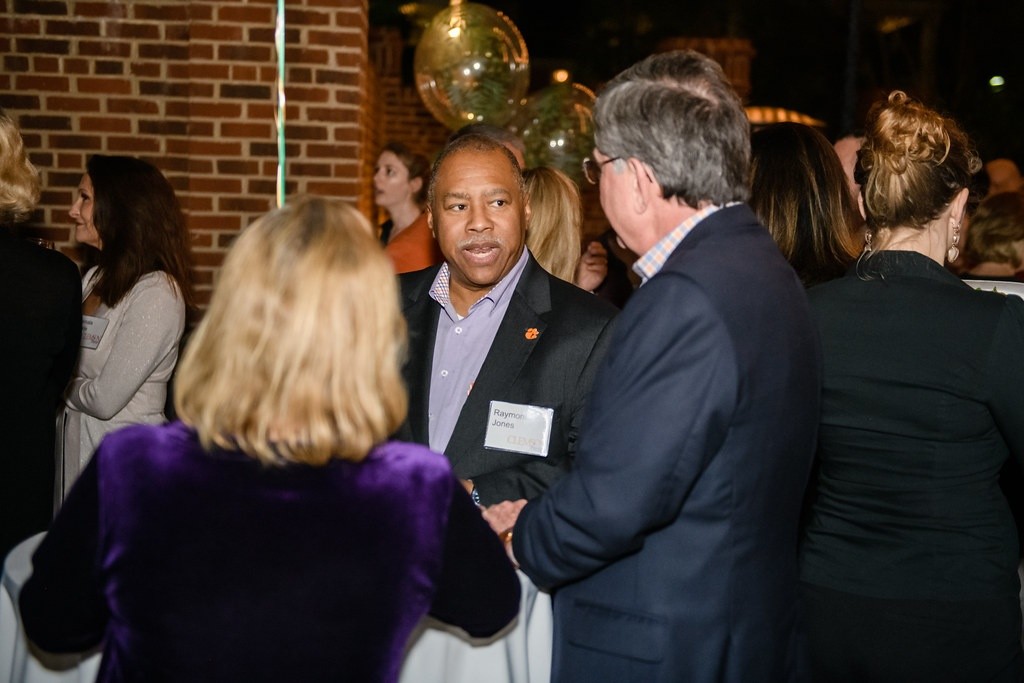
[582,156,624,184]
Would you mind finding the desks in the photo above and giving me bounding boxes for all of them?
[3,529,556,683]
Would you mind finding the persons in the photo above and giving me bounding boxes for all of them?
[748,87,1024,683]
[0,107,196,583]
[370,144,610,294]
[478,46,814,683]
[18,193,523,683]
[388,135,624,509]
